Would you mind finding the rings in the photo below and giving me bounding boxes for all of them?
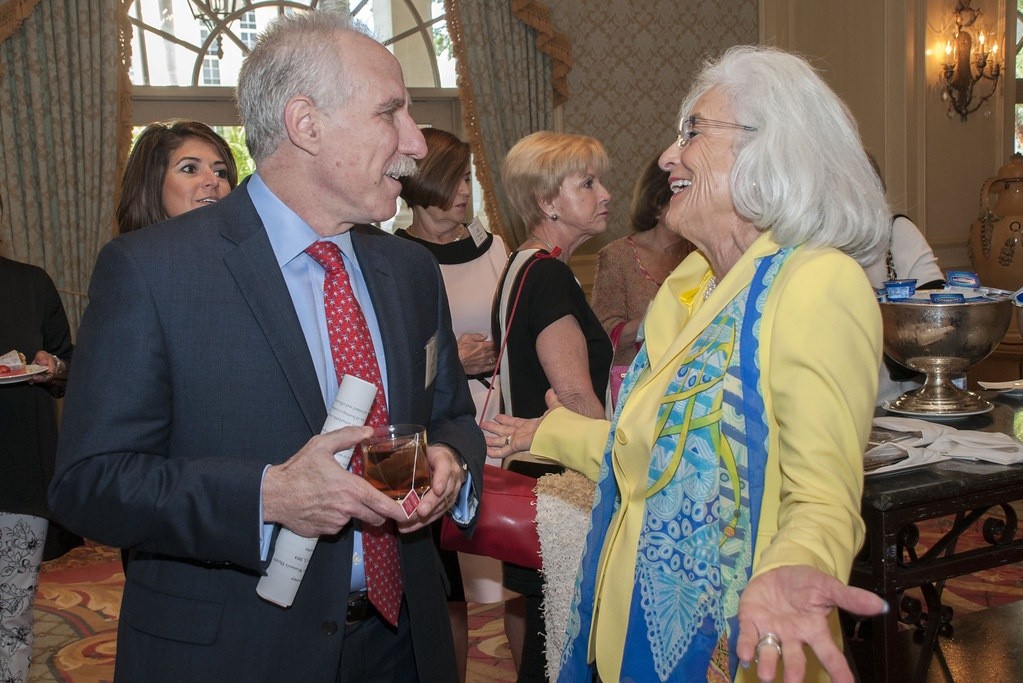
[445,497,448,507]
[505,435,512,447]
[490,358,493,365]
[754,633,782,665]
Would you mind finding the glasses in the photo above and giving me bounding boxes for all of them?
[675,116,760,150]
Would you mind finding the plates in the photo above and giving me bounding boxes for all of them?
[868,418,960,445]
[863,447,949,480]
[976,380,1023,401]
[883,402,994,423]
[0,365,50,385]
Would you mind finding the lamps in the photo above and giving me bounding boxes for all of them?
[925,1,1005,123]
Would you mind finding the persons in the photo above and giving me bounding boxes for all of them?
[482,44,895,683]
[863,148,966,408]
[47,8,488,683]
[0,193,73,683]
[393,128,524,683]
[588,152,698,367]
[115,120,239,235]
[490,131,616,683]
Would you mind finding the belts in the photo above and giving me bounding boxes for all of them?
[345,593,377,630]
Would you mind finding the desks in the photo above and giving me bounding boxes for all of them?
[838,387,1023,683]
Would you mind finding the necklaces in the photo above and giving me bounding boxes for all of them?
[453,223,465,242]
[529,234,553,251]
[668,252,682,259]
[703,278,716,300]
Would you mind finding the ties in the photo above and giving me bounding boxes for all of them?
[305,243,402,632]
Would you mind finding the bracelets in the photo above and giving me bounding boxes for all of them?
[53,354,60,376]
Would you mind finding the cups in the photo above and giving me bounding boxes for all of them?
[360,422,432,505]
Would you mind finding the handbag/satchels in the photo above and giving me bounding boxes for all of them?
[879,212,971,382]
[607,322,644,413]
[437,462,548,571]
